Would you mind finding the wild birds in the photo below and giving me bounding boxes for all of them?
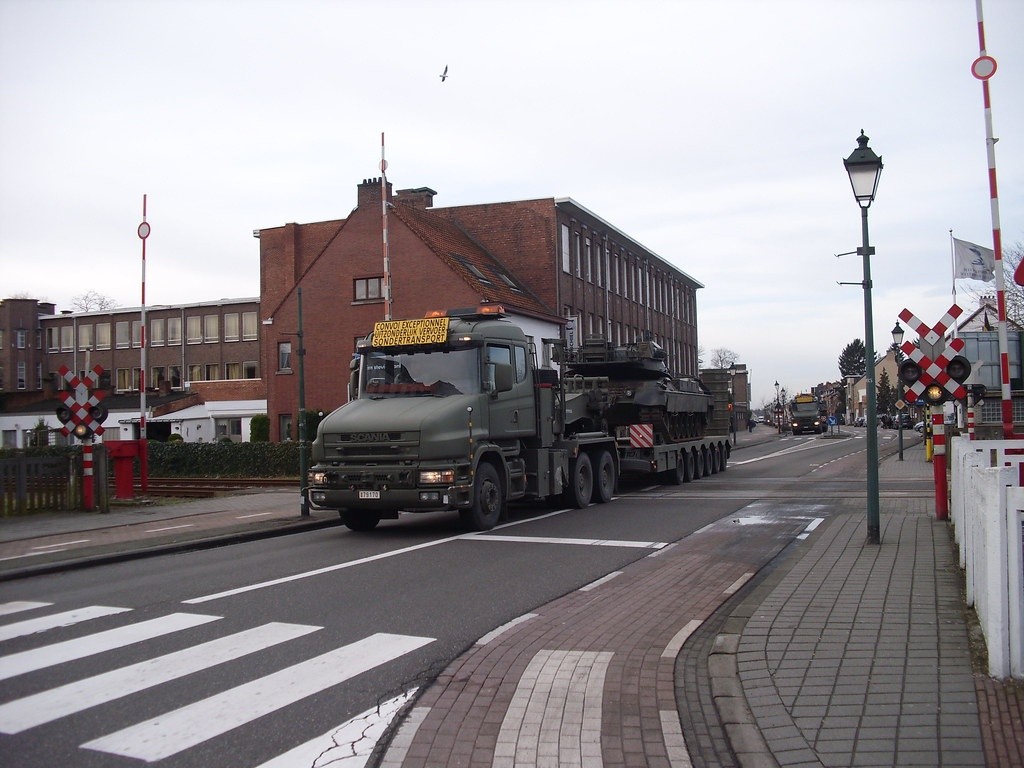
[440,65,448,82]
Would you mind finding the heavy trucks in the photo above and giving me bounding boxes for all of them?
[302,296,738,534]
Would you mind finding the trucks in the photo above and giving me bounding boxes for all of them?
[789,393,829,434]
[759,415,765,423]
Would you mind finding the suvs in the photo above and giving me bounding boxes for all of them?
[892,413,916,430]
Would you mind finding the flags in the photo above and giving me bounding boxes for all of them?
[953,239,996,283]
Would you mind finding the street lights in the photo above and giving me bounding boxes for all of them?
[780,387,786,431]
[841,125,886,546]
[891,318,906,461]
[774,380,781,434]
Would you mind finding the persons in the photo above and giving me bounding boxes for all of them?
[881,415,893,429]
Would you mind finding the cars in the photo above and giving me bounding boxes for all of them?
[914,417,956,432]
[854,417,867,426]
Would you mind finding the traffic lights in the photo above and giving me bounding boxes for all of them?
[55,405,109,441]
[899,355,973,406]
